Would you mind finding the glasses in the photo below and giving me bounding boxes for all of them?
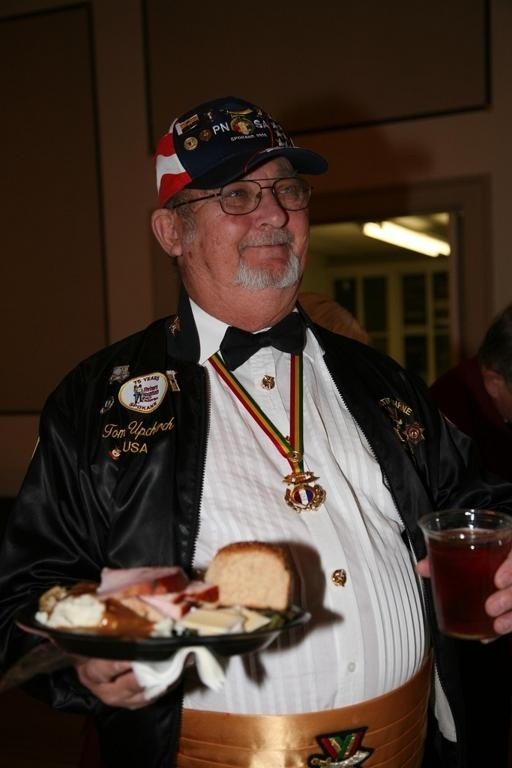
[171,176,312,217]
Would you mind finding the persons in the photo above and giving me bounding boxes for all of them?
[428,308,511,767]
[1,91,487,767]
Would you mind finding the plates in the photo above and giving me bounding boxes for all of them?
[17,604,312,661]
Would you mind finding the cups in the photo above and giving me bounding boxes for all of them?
[418,508,511,640]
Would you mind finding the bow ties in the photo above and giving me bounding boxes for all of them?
[216,312,309,373]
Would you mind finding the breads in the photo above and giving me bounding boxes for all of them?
[34,593,175,641]
[203,542,297,611]
[97,566,219,620]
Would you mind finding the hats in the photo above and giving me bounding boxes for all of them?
[152,95,329,209]
